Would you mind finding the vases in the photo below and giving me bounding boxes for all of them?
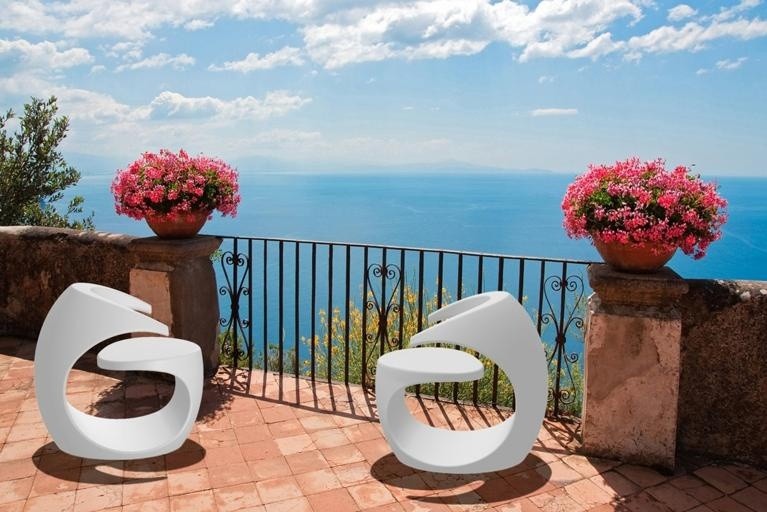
[590,234,679,273]
[145,206,211,239]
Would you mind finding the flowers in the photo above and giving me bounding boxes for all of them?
[111,149,241,224]
[561,155,728,260]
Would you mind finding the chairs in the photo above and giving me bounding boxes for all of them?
[34,283,205,460]
[374,291,549,476]
